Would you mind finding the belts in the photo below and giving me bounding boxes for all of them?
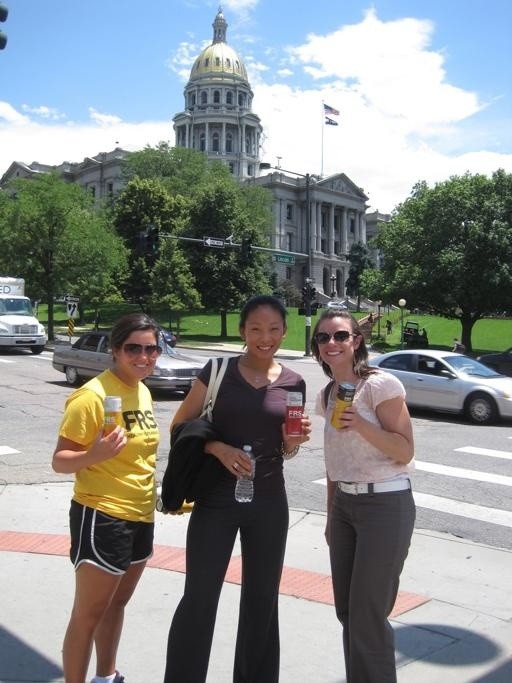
[336,479,411,495]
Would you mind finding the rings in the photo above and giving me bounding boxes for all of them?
[232,462,239,468]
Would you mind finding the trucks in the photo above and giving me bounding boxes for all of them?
[0,276,47,352]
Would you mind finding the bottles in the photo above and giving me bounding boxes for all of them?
[157,498,195,513]
[330,382,356,428]
[103,396,122,437]
[235,445,255,502]
[285,391,304,437]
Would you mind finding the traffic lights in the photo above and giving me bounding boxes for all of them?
[298,309,305,315]
[302,287,308,302]
[243,238,252,258]
[310,288,316,301]
[311,308,317,315]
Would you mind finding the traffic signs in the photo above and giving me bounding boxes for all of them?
[66,303,77,317]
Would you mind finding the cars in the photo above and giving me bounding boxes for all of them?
[54,328,206,393]
[369,349,512,425]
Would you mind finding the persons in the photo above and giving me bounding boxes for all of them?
[162,294,310,683]
[52,311,158,683]
[313,309,416,683]
[452,338,465,354]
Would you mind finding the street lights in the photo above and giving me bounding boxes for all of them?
[398,299,409,347]
[376,300,382,341]
[331,274,336,296]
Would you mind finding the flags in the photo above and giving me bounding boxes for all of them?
[323,117,339,126]
[323,103,341,115]
